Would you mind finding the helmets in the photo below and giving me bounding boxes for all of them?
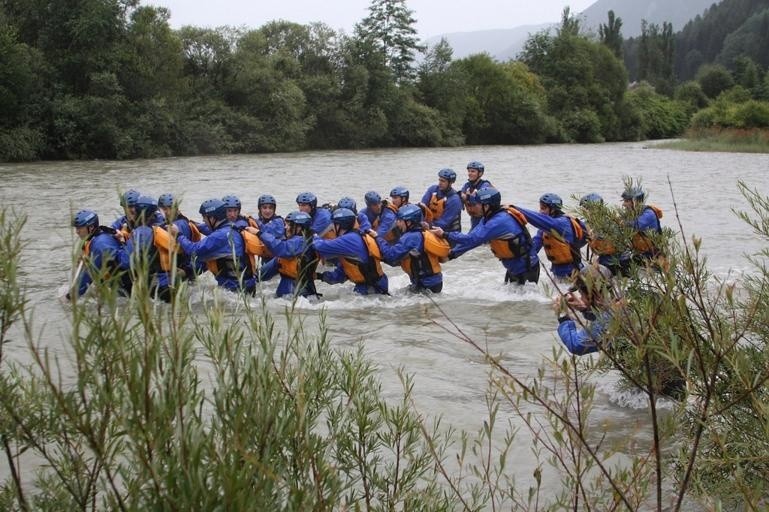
[330,197,356,222]
[467,161,484,174]
[397,203,421,220]
[365,191,381,204]
[475,187,501,207]
[438,168,456,181]
[390,187,409,198]
[580,193,603,208]
[540,192,562,207]
[258,194,276,207]
[119,189,179,210]
[297,192,317,205]
[285,211,312,227]
[72,209,98,228]
[567,263,614,295]
[199,195,241,214]
[621,189,643,200]
